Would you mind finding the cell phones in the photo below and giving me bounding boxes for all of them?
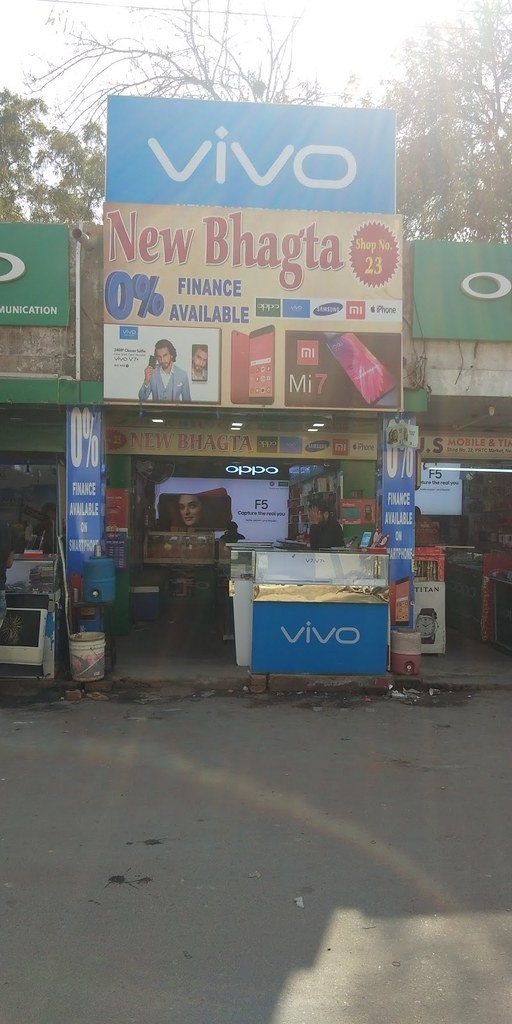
[149,355,157,369]
[157,487,232,532]
[191,344,208,381]
[325,333,397,404]
[230,324,275,405]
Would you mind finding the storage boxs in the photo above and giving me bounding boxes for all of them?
[131,585,160,621]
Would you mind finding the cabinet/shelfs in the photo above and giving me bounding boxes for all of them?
[445,558,512,657]
[288,468,345,542]
[130,530,392,673]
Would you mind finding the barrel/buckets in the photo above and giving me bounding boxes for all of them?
[69,632,106,682]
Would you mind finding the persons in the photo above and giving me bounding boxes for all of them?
[220,522,245,543]
[308,500,346,552]
[193,346,207,380]
[32,503,57,554]
[0,521,14,627]
[415,506,438,546]
[177,494,210,528]
[139,339,191,401]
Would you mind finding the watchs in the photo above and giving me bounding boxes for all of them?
[415,608,439,644]
